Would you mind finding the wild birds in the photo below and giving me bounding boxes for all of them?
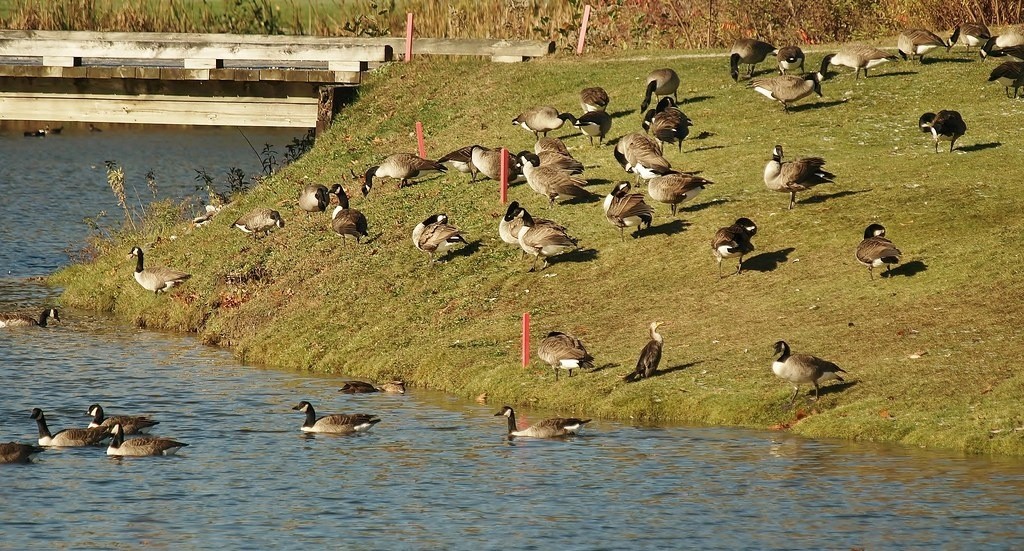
[128,246,193,299]
[291,401,381,434]
[856,224,902,283]
[0,308,61,328]
[377,381,407,394]
[0,442,46,464]
[362,152,448,199]
[406,20,1024,273]
[230,206,285,242]
[83,404,160,435]
[622,321,665,384]
[23,122,102,138]
[100,423,189,457]
[30,408,112,446]
[772,340,846,406]
[713,219,756,281]
[338,381,385,395]
[492,406,592,437]
[299,183,368,246]
[537,332,595,382]
